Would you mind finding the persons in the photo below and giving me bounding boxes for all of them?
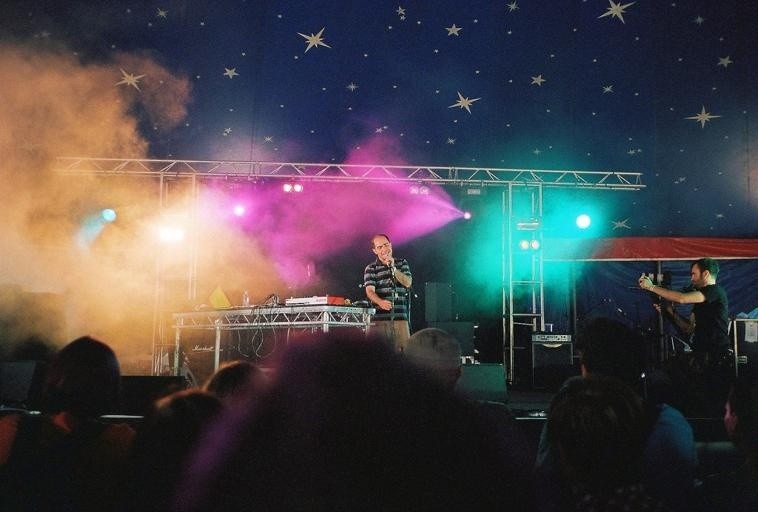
[363,234,414,354]
[2,304,756,510]
[639,257,731,381]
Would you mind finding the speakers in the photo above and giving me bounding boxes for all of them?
[733,318,758,377]
[454,364,508,402]
[428,321,474,356]
[424,282,451,322]
[532,342,573,392]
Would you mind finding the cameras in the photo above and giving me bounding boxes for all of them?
[641,272,671,285]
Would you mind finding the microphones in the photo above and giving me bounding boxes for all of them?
[387,260,393,268]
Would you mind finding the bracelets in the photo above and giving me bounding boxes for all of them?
[649,284,655,291]
[391,266,397,271]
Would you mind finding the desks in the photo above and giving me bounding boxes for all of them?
[170,305,376,381]
